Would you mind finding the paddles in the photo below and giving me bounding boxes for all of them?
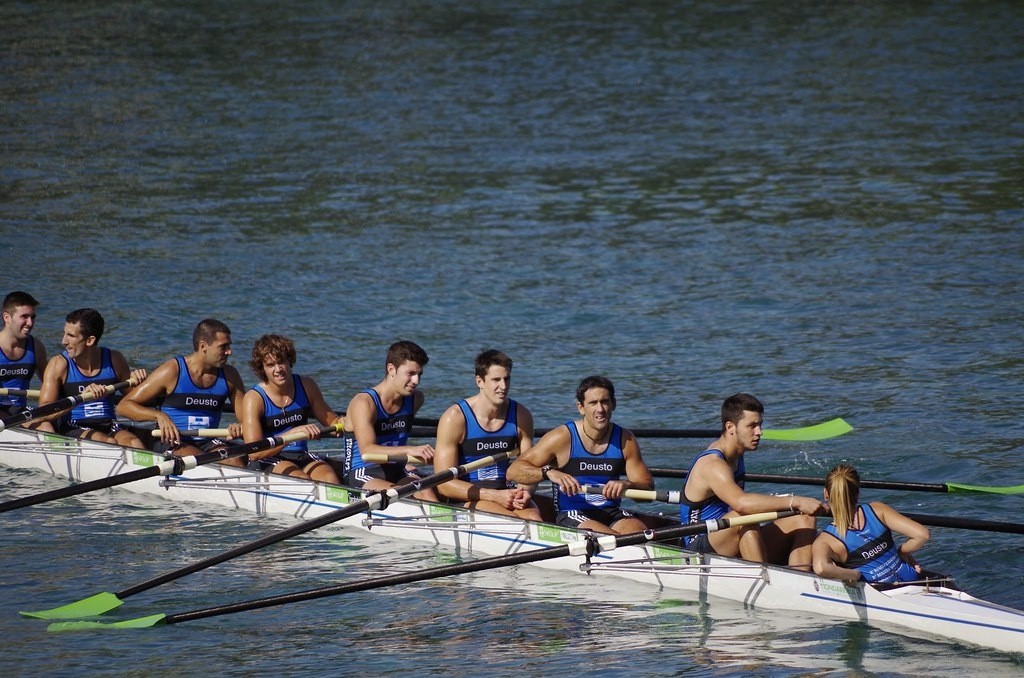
[360,452,1024,496]
[18,447,521,620]
[0,423,344,512]
[0,387,440,427]
[558,481,1024,535]
[0,377,136,432]
[46,509,800,632]
[151,413,854,444]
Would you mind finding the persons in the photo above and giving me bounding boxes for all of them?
[0,291,54,433]
[506,376,655,535]
[343,341,439,502]
[242,334,346,485]
[680,393,830,572]
[812,465,960,590]
[39,308,148,449]
[115,318,246,468]
[433,350,543,521]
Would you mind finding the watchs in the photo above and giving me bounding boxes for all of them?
[542,465,554,480]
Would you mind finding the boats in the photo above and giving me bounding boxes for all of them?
[0,426,1024,655]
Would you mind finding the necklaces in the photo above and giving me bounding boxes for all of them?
[265,381,291,416]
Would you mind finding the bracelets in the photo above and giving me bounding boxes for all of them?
[789,494,794,512]
